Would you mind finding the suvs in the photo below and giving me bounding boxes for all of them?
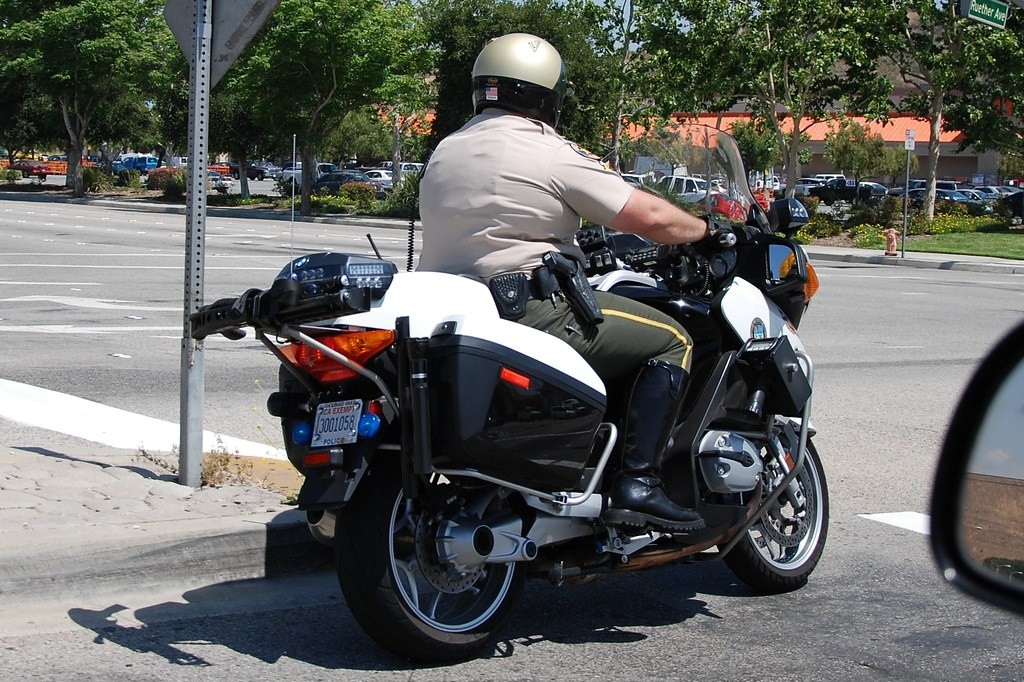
[888,179,971,196]
[779,179,827,196]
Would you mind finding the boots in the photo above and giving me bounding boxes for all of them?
[604,357,705,529]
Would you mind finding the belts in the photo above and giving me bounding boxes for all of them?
[526,278,542,301]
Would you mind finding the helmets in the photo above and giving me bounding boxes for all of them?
[471,33,568,131]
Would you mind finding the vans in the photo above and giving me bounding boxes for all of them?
[816,174,846,181]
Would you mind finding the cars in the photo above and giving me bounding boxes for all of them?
[313,171,384,199]
[175,157,188,169]
[366,170,404,192]
[208,161,239,175]
[900,184,1023,204]
[12,151,102,163]
[206,170,232,193]
[620,170,785,219]
[995,191,1024,223]
[3,160,50,178]
[358,161,423,174]
[846,180,888,196]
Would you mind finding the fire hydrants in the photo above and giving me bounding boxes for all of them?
[883,228,901,257]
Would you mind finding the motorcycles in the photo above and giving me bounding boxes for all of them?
[188,123,829,657]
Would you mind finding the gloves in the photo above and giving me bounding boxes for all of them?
[694,215,733,254]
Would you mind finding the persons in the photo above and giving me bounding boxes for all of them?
[417,32,738,529]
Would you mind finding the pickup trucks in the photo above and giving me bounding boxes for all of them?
[112,156,159,175]
[281,163,340,184]
[807,178,871,204]
[229,159,283,181]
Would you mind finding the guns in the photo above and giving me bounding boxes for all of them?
[542,249,606,326]
